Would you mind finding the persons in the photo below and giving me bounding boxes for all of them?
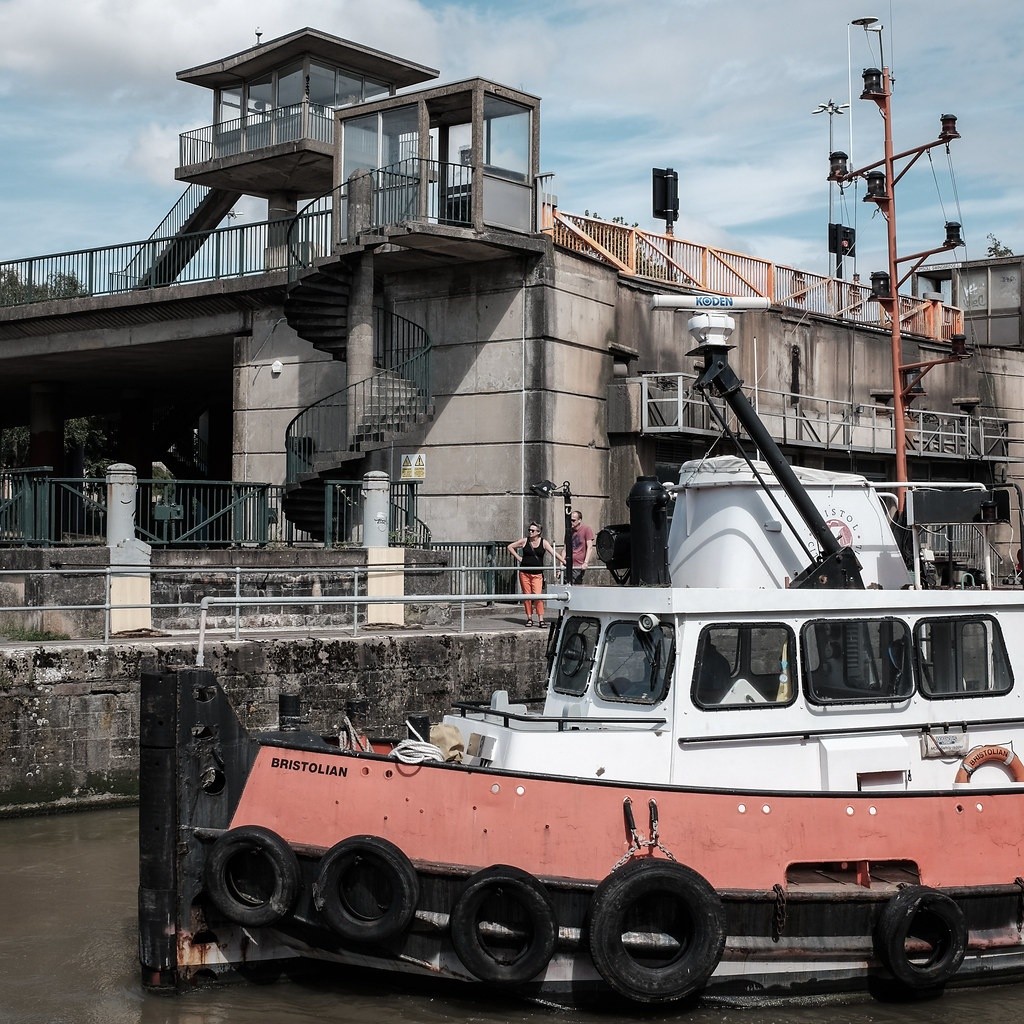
[507,522,566,628]
[557,511,594,585]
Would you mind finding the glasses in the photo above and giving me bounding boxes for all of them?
[528,529,535,533]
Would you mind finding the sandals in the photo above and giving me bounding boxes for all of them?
[525,619,533,627]
[539,621,548,628]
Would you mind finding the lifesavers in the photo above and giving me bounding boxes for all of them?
[206,825,303,925]
[588,857,729,1005]
[955,746,1024,783]
[874,884,970,984]
[314,834,422,943]
[449,865,559,982]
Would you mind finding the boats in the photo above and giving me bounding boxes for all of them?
[138,297,1024,1008]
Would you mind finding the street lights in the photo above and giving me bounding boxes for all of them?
[826,17,965,511]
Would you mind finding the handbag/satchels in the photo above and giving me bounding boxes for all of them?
[542,576,545,589]
